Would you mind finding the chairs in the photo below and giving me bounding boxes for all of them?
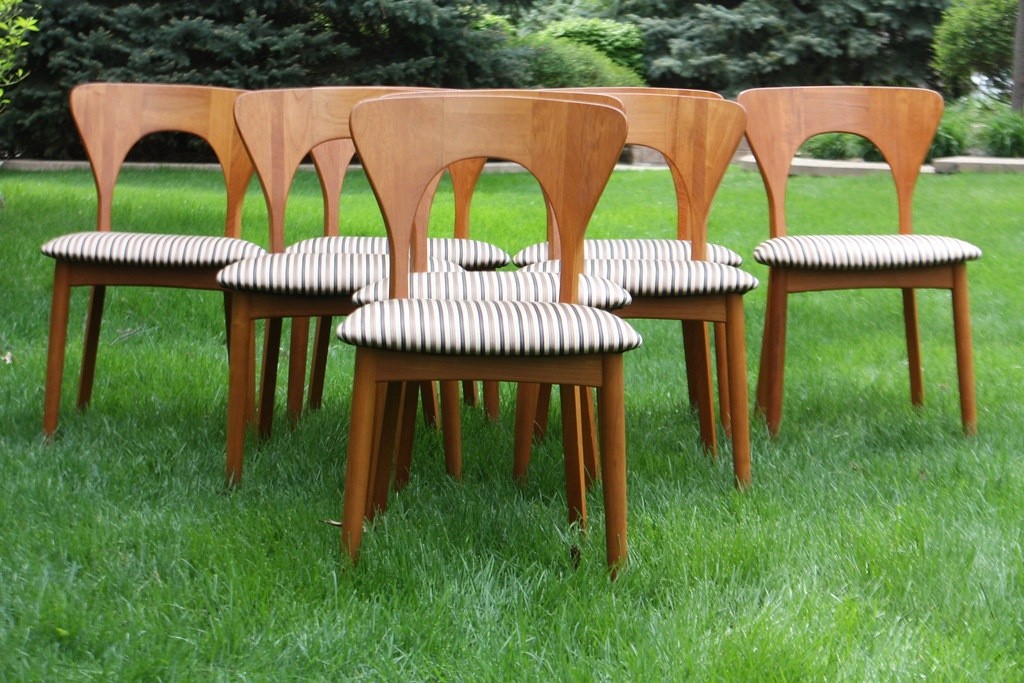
[41,83,983,580]
